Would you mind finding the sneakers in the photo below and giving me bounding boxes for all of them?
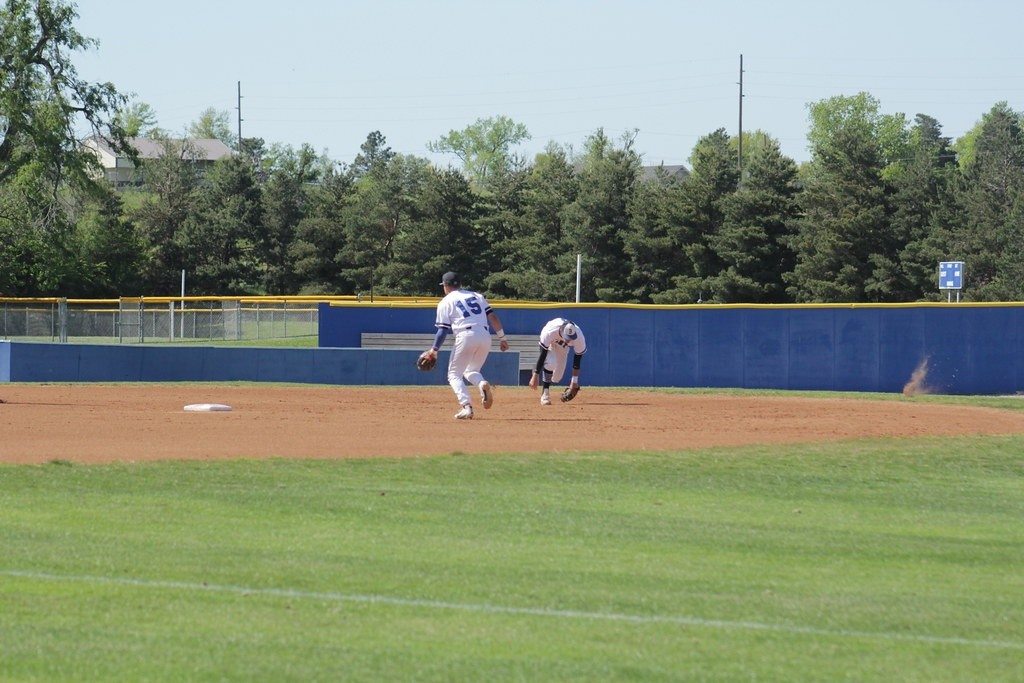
[540,388,551,406]
[479,380,494,410]
[454,405,474,420]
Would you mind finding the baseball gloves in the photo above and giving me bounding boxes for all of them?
[416,350,437,372]
[560,384,580,403]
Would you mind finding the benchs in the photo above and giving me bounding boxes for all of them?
[361,332,547,386]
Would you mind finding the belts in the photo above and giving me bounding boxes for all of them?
[466,326,487,331]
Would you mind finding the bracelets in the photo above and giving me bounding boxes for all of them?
[497,328,505,341]
[571,376,579,384]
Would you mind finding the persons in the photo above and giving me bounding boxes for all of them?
[416,272,510,420]
[529,318,587,405]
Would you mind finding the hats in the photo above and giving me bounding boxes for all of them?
[560,323,578,340]
[438,271,460,287]
